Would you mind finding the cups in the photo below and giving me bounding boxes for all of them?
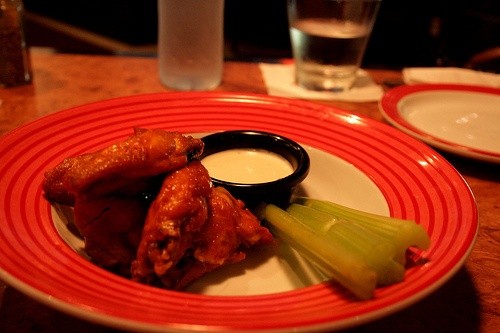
[157,0,224,91]
[287,0,381,92]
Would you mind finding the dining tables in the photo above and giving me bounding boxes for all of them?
[0,55,500,333]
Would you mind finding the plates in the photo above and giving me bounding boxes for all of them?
[377,83,500,164]
[401,67,500,89]
[0,91,477,333]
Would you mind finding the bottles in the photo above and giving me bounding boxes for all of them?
[0,0,33,87]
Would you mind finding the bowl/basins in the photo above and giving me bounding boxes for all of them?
[191,131,310,223]
